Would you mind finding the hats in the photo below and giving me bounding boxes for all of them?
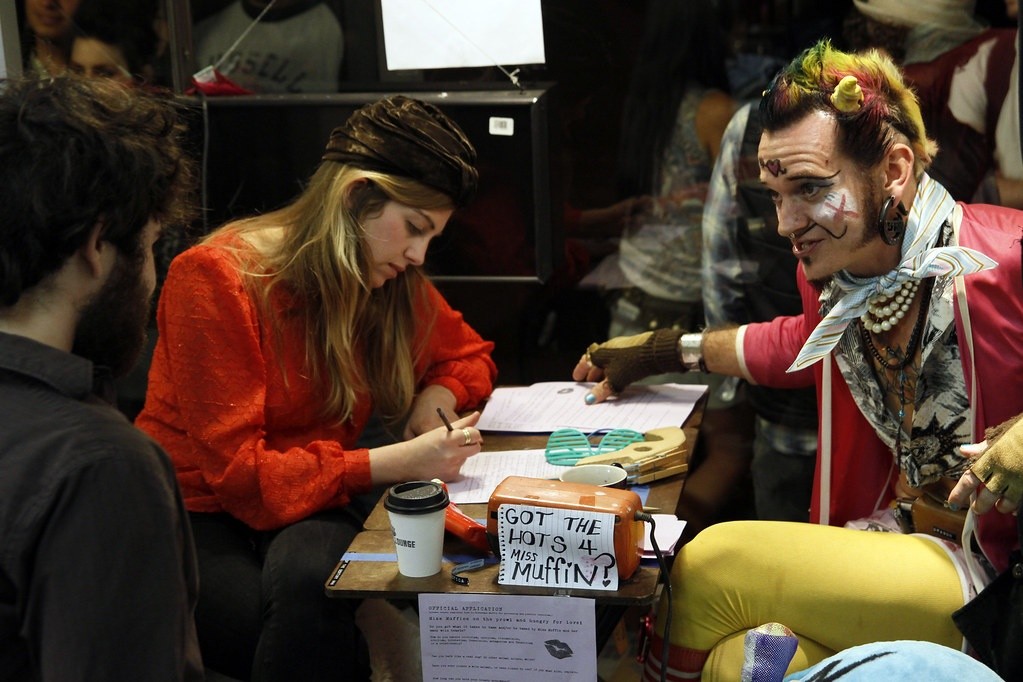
[322,95,480,208]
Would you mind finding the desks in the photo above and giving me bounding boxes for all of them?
[323,386,711,682]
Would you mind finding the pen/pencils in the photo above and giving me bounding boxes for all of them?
[436,408,454,431]
[642,506,661,513]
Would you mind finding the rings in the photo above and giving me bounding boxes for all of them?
[464,428,472,445]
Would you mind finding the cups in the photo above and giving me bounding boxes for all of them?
[559,463,628,490]
[383,481,450,578]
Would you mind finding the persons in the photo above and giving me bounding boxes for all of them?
[0,1,500,682]
[840,0,1022,209]
[572,37,1023,682]
[563,0,821,535]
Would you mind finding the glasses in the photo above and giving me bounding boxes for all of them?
[545,428,644,466]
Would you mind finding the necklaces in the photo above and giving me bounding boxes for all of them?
[856,279,936,474]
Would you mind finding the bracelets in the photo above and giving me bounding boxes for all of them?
[680,332,710,375]
